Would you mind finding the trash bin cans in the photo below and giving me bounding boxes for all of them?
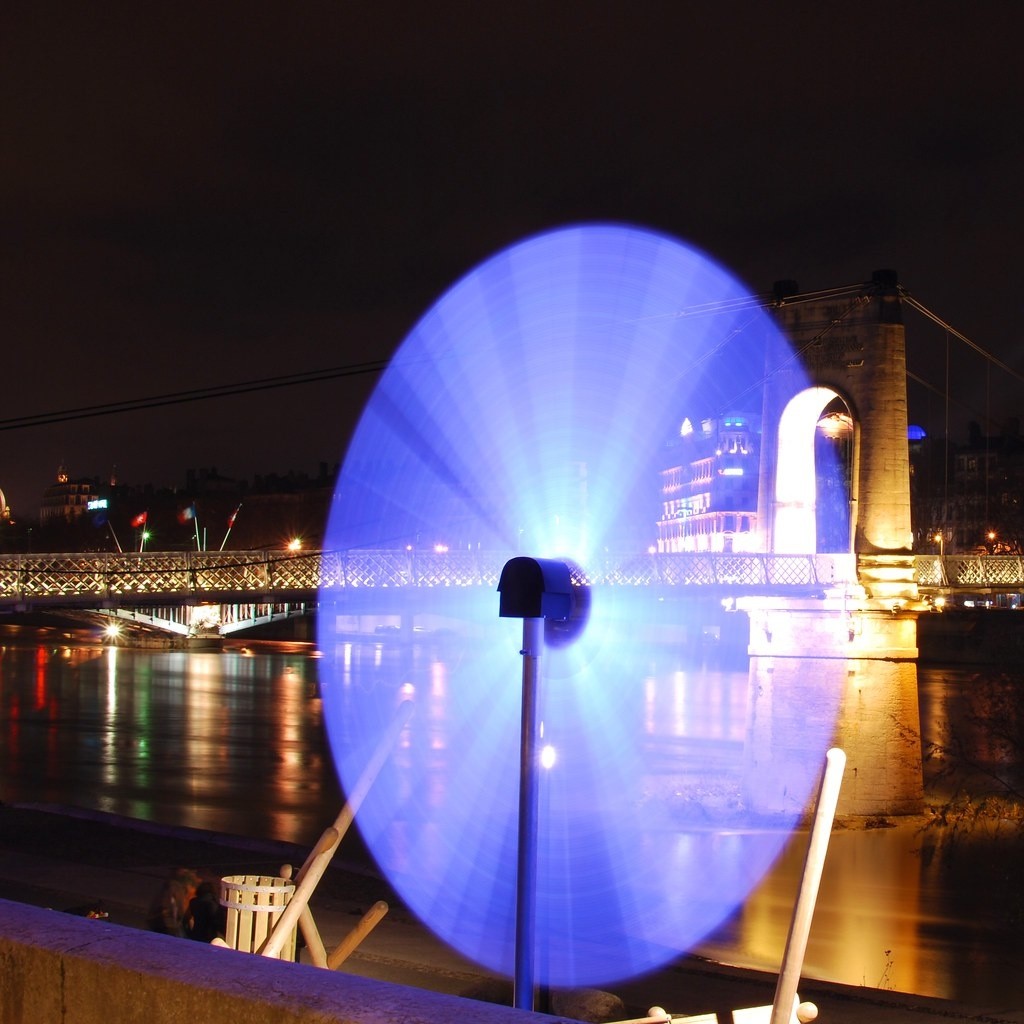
[219,875,302,963]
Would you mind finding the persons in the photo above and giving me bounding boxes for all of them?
[155,869,226,943]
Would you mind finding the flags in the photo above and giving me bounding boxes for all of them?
[178,503,196,522]
[131,512,147,526]
[227,505,239,528]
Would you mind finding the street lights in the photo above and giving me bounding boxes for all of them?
[143,532,150,550]
[989,530,995,555]
[935,535,944,555]
